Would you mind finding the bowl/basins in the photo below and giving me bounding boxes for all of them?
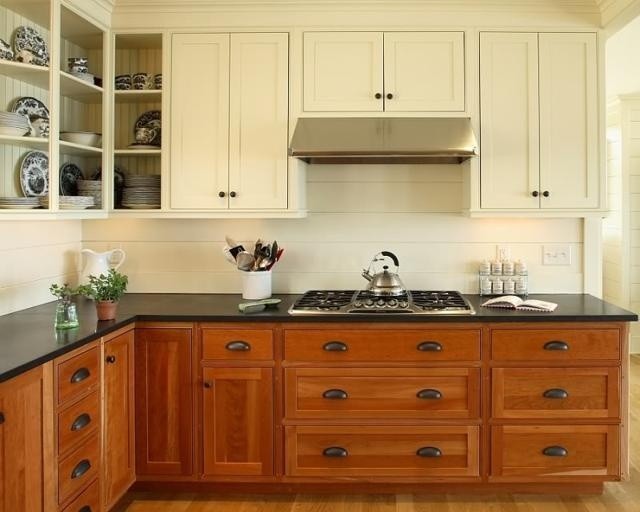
[59,131,102,146]
[115,72,162,89]
[67,56,94,84]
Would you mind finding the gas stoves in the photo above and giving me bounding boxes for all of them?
[288,289,476,316]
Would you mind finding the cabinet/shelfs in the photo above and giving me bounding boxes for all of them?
[301,29,466,117]
[0,1,113,220]
[476,29,598,209]
[108,15,164,215]
[169,33,289,212]
[0,362,55,511]
[51,330,139,512]
[135,328,198,480]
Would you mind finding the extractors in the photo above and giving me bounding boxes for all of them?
[288,117,478,166]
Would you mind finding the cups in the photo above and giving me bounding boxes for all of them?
[242,270,272,300]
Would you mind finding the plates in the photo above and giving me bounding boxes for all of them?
[59,160,101,210]
[128,109,162,149]
[115,167,161,208]
[0,150,49,210]
[0,25,48,68]
[0,96,50,138]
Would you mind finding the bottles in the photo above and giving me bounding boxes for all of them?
[479,253,529,298]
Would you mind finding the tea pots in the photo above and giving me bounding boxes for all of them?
[80,248,126,299]
[361,251,406,297]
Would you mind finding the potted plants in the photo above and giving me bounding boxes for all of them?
[45,267,130,330]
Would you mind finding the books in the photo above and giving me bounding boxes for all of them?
[480,294,559,313]
